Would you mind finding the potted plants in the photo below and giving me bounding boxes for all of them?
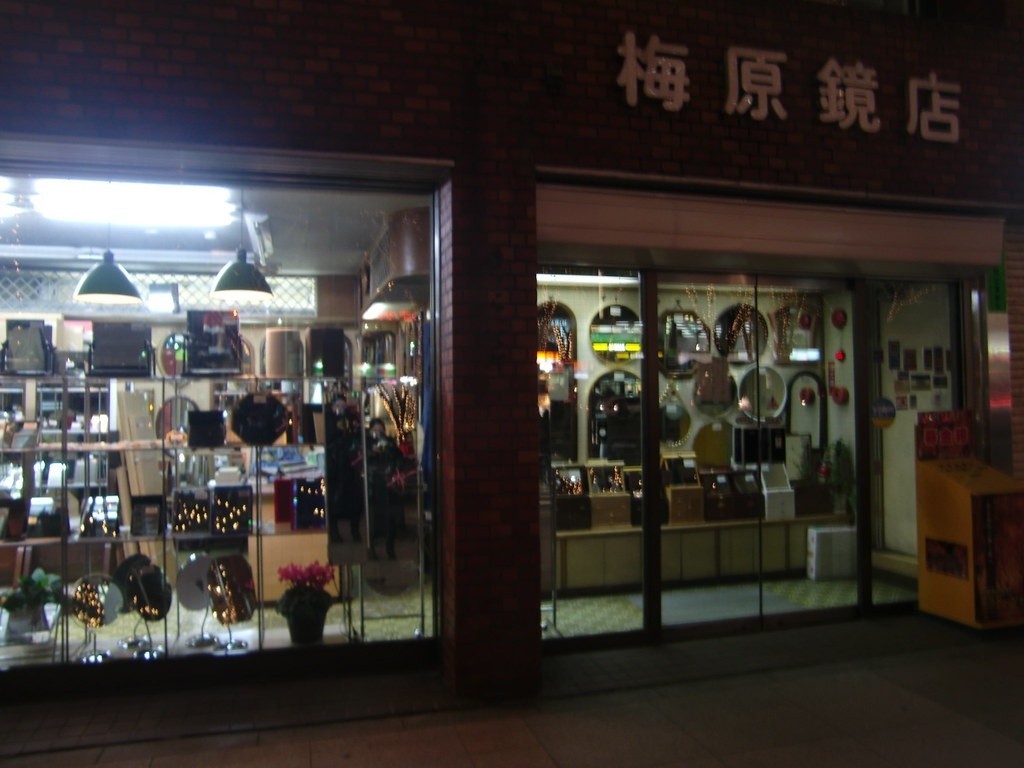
[3,567,62,647]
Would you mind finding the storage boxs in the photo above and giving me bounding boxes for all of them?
[807,527,856,582]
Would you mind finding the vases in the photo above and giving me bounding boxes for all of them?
[280,585,331,642]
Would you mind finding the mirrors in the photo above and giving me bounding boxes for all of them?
[322,389,372,563]
[156,329,193,388]
[538,298,823,496]
[154,397,203,462]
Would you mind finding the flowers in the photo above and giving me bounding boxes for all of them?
[279,559,335,588]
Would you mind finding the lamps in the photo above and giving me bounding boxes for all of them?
[212,188,275,302]
[74,250,142,307]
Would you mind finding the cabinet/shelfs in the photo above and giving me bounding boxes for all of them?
[1,369,371,663]
[554,467,856,594]
[358,210,429,337]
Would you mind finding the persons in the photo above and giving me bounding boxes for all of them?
[324,395,404,560]
